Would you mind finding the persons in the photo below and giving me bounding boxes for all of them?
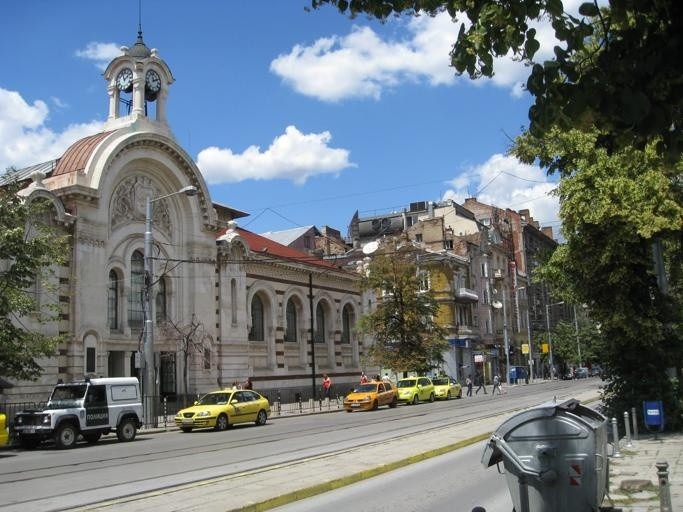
[475,373,489,394]
[382,373,391,382]
[492,373,500,394]
[241,376,252,389]
[321,374,331,400]
[231,380,238,389]
[360,371,367,384]
[465,373,472,396]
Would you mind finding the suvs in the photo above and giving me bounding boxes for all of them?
[14,374,145,447]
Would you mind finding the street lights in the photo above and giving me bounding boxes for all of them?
[501,286,527,385]
[547,301,564,380]
[142,184,198,427]
[416,249,447,279]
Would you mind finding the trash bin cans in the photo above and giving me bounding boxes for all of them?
[480,398,609,512]
[295,391,302,402]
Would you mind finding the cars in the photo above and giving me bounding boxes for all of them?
[343,381,398,414]
[395,376,435,403]
[174,390,270,433]
[429,375,463,400]
[563,366,603,380]
